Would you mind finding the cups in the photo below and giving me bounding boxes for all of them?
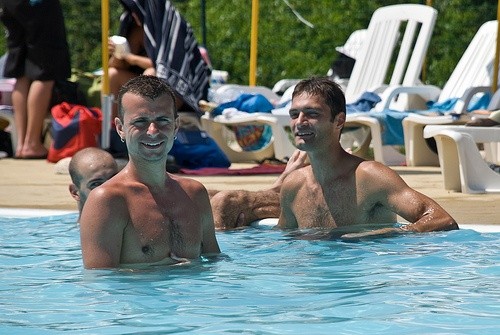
[110,36,127,60]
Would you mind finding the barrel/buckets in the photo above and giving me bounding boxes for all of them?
[209,70,228,89]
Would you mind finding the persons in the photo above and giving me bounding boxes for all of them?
[0,0,72,159]
[80,74,223,270]
[107,1,211,128]
[67,147,309,234]
[269,77,459,239]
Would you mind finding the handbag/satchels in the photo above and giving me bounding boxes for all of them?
[47,101,102,163]
[169,130,232,169]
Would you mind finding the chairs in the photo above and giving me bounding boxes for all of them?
[422,123,499,195]
[292,4,439,170]
[352,20,499,190]
[202,76,328,166]
